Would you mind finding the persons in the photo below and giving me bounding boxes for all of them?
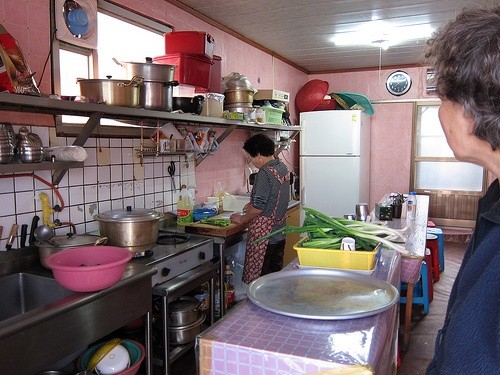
[415,0,500,375]
[230,133,291,285]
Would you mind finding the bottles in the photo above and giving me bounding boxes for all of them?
[177,185,194,226]
[406,192,417,219]
[214,266,235,310]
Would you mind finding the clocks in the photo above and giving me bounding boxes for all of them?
[386,70,412,97]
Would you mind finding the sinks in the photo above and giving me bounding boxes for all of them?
[0,272,76,323]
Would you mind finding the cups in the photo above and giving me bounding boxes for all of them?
[391,204,402,218]
[207,197,219,209]
[42,146,87,162]
[355,205,367,221]
[340,237,356,251]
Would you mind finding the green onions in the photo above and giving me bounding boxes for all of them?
[251,207,410,256]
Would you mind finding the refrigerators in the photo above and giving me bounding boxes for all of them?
[298,110,371,238]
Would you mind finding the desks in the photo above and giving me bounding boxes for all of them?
[368,193,430,350]
[187,246,400,375]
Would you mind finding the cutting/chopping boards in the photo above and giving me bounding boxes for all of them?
[184,212,245,237]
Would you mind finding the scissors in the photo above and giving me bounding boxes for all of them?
[167,161,178,191]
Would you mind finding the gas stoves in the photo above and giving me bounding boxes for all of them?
[129,231,214,287]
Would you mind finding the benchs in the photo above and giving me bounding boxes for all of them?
[416,192,484,228]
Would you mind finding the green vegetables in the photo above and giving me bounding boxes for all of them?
[199,217,231,227]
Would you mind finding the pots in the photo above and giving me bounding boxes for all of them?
[93,207,165,253]
[34,233,109,271]
[159,297,207,345]
[76,62,203,118]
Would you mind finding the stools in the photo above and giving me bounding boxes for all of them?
[400,221,444,315]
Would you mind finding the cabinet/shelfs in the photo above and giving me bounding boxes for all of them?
[0,92,303,186]
[144,256,220,375]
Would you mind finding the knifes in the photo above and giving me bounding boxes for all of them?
[0,216,40,251]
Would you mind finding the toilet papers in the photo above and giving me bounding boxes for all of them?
[207,196,219,208]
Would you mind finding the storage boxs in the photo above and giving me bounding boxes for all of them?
[154,30,291,124]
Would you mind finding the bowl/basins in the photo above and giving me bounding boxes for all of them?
[0,122,44,164]
[224,89,256,116]
[43,246,135,293]
[295,79,338,112]
[77,338,146,375]
